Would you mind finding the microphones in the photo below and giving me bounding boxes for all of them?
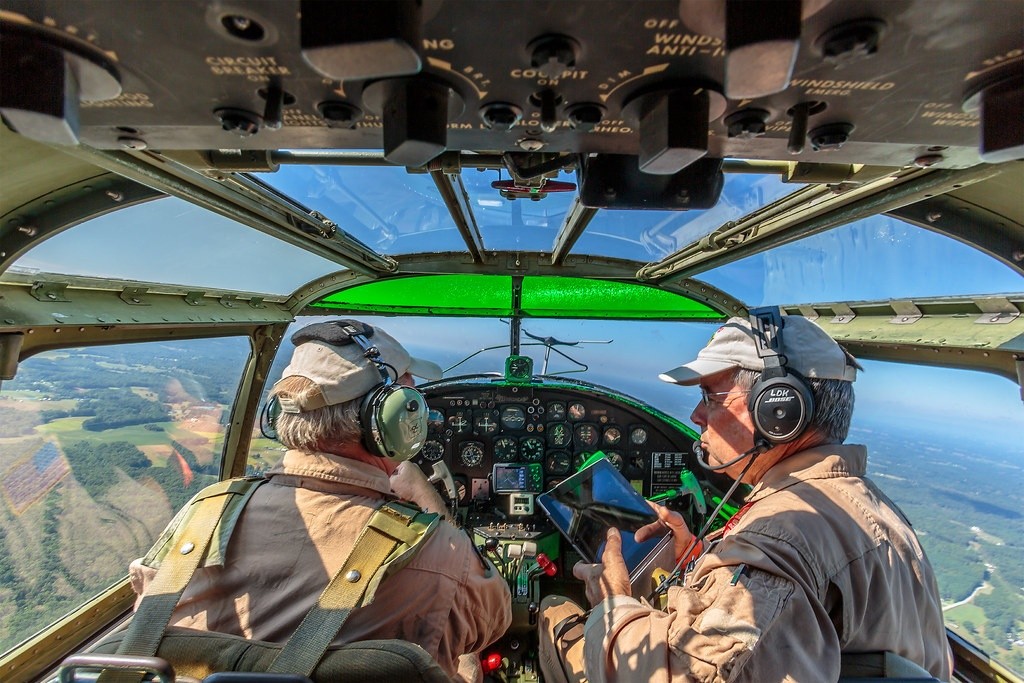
[692,440,769,470]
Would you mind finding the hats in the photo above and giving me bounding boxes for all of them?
[274,324,443,414]
[659,316,858,386]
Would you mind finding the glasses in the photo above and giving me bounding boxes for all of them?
[702,385,750,407]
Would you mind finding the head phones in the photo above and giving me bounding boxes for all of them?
[267,321,429,463]
[744,306,815,453]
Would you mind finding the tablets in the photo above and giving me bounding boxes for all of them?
[536,457,673,588]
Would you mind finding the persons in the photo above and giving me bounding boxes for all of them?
[128,322,511,683]
[539,317,955,683]
